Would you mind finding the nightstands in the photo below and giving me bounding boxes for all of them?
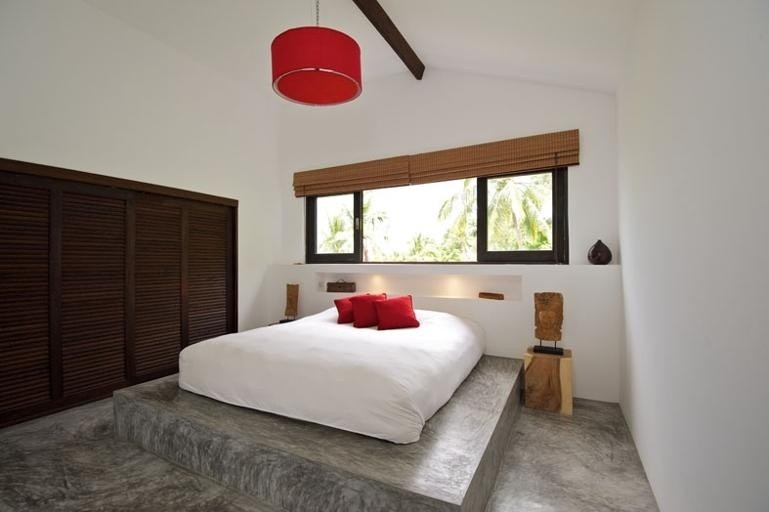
[524,345,574,419]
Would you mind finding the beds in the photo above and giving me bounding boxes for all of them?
[174,293,488,447]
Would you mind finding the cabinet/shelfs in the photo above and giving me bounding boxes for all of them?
[127,179,183,390]
[52,165,129,410]
[0,155,52,428]
[178,191,238,351]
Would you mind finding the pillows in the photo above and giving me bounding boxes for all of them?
[334,297,353,324]
[375,294,420,329]
[349,294,386,328]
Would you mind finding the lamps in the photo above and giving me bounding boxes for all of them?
[531,291,564,356]
[285,282,299,320]
[269,1,362,107]
